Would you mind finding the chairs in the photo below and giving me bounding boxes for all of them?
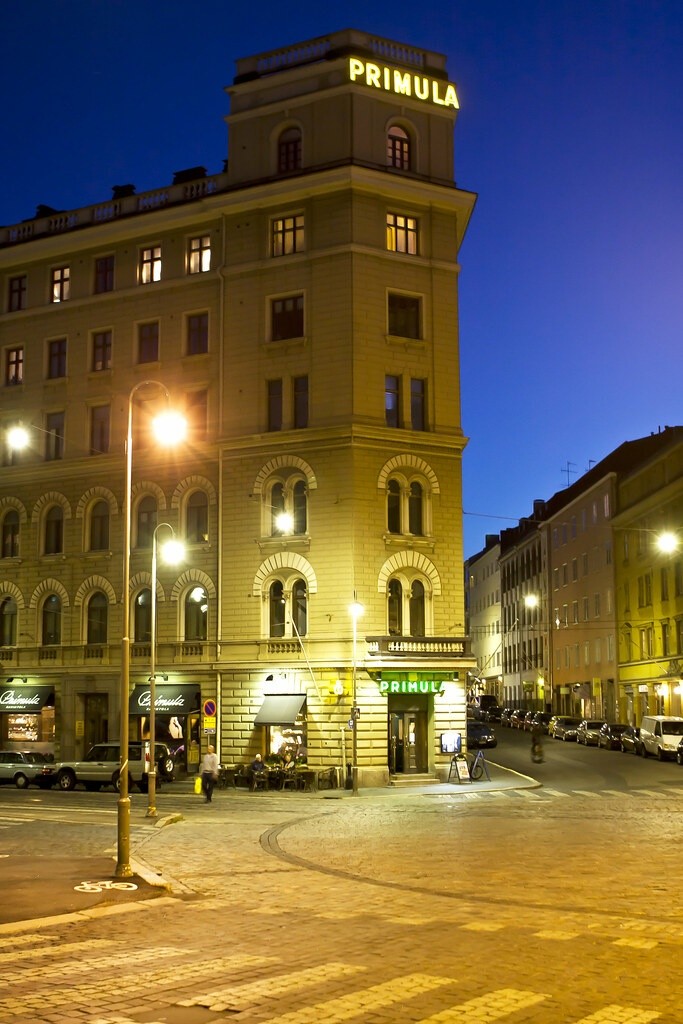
[218,763,339,793]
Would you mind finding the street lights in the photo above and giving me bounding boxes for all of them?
[111,381,194,878]
[347,600,366,797]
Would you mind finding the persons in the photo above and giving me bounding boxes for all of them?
[198,745,219,804]
[249,754,265,791]
[271,755,295,789]
[531,726,542,755]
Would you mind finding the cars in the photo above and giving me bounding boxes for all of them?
[464,699,643,752]
[1,749,53,788]
[675,739,683,765]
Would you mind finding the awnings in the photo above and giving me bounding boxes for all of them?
[254,695,306,727]
[129,685,197,716]
[0,687,54,713]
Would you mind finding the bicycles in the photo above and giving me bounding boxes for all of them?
[469,750,484,780]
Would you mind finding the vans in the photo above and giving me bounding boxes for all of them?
[640,715,683,759]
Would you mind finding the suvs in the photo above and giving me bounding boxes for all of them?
[56,741,174,793]
